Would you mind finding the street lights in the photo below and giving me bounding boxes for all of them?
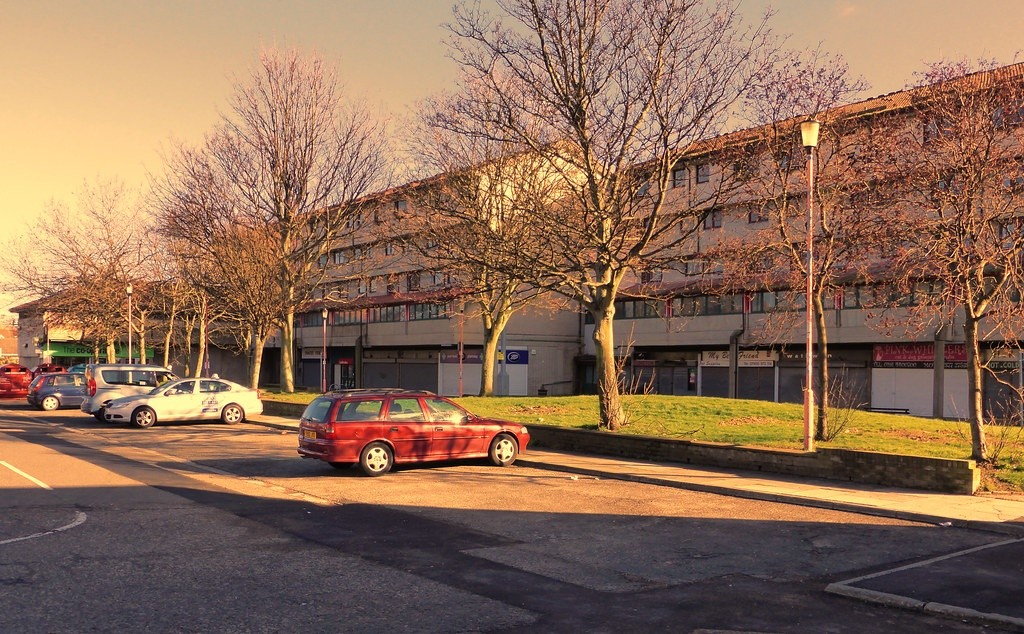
[322,307,328,392]
[799,120,823,452]
[126,283,133,384]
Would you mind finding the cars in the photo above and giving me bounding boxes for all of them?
[104,377,264,427]
[27,372,86,410]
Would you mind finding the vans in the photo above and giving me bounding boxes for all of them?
[80,362,184,420]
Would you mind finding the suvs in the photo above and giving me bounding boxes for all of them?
[297,387,531,477]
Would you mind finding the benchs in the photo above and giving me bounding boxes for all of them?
[863,407,910,415]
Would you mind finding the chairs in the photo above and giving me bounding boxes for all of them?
[391,404,402,413]
[404,409,417,421]
